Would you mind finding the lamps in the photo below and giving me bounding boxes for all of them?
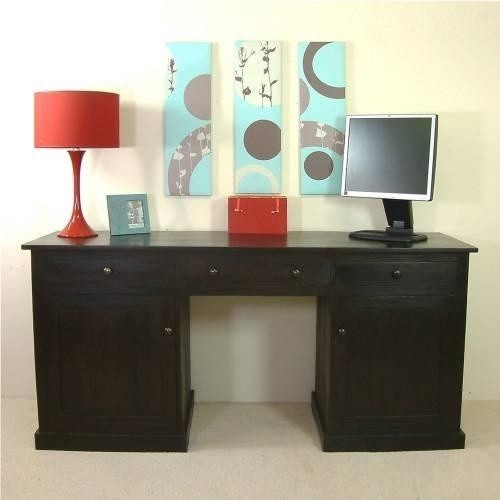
[35,91,120,239]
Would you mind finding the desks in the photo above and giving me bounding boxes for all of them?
[22,230,479,452]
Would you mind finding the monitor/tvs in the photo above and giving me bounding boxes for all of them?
[341,115,438,243]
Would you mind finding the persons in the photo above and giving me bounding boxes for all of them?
[130,204,142,224]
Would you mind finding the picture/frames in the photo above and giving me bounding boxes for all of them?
[107,194,152,236]
